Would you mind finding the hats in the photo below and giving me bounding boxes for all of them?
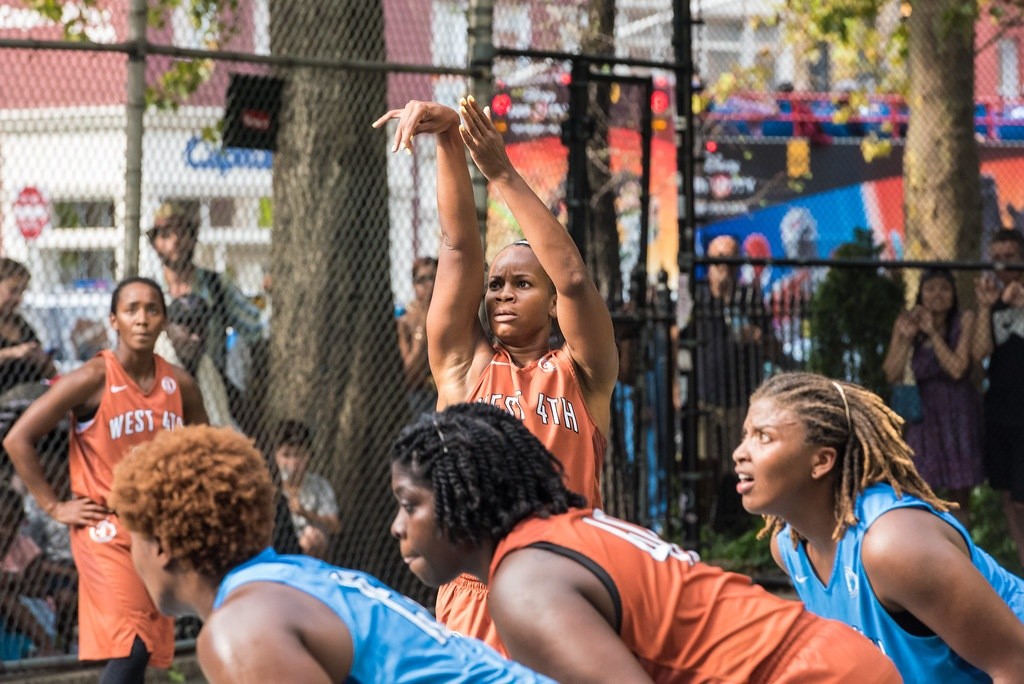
[146,202,198,241]
[167,295,209,324]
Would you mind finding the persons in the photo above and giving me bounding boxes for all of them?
[733,373,1024,684]
[5,276,208,683]
[371,93,620,660]
[3,191,1021,654]
[109,425,559,683]
[388,402,903,684]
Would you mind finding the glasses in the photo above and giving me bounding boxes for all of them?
[413,274,435,284]
